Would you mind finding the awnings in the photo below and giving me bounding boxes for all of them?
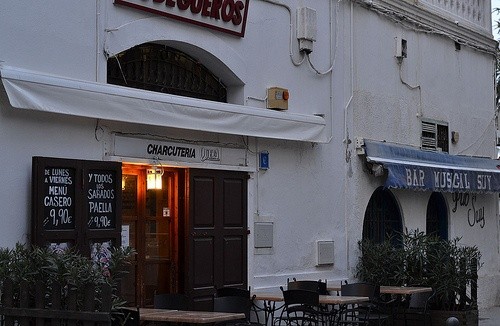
[362,138,500,193]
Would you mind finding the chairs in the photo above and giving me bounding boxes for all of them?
[176,276,439,326]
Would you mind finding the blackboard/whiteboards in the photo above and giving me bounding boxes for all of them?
[29,156,122,239]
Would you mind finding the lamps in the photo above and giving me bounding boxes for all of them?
[146,154,165,191]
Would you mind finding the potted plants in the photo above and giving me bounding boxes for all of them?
[352,226,487,326]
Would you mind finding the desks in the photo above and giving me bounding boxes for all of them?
[114,304,247,326]
